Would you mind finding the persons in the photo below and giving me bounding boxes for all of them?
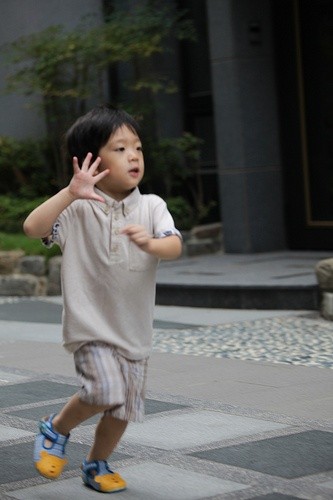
[17,105,187,492]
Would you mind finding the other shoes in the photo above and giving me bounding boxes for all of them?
[32,412,71,480]
[79,458,128,493]
